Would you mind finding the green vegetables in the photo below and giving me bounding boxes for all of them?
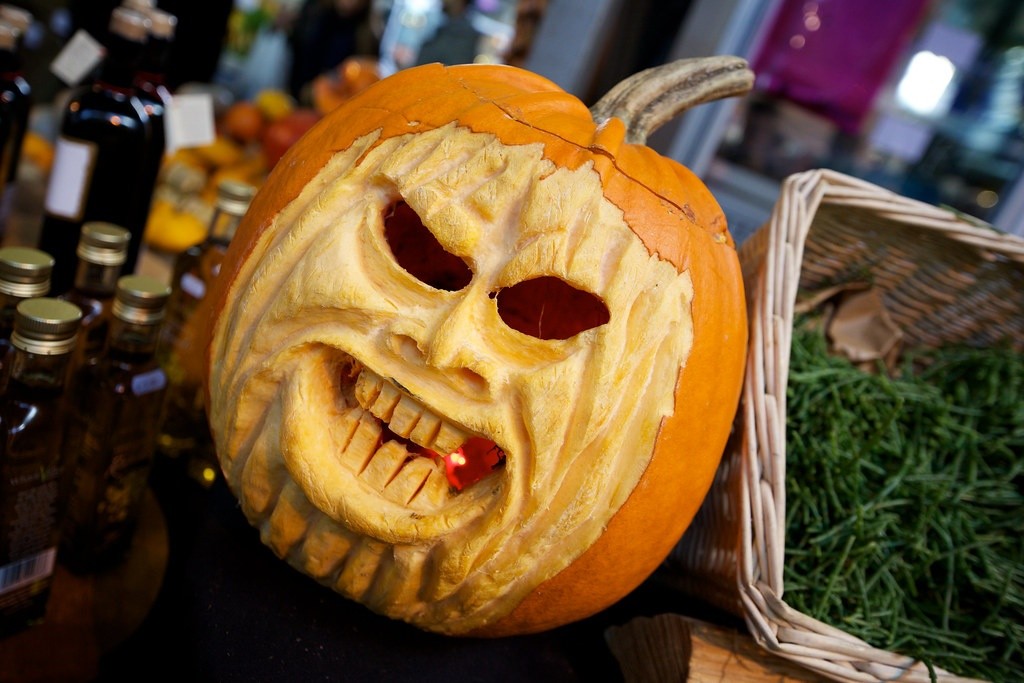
[786,323,1024,683]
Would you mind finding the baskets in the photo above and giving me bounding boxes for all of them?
[661,169,1024,683]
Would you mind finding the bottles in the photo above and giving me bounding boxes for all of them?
[61,221,130,412]
[0,0,178,292]
[157,180,255,365]
[64,274,168,575]
[0,246,55,381]
[0,298,84,633]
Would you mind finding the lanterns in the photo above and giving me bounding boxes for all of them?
[169,51,758,640]
